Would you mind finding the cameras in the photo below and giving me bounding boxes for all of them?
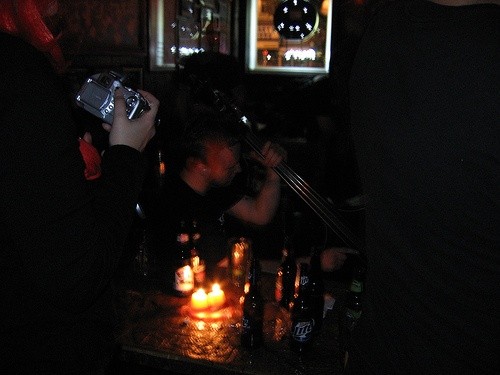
[72,70,149,128]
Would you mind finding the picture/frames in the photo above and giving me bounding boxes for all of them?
[147,0,243,74]
[244,0,333,77]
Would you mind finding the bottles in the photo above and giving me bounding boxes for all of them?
[290,263,317,352]
[171,215,209,298]
[340,263,364,321]
[306,245,325,336]
[239,257,264,351]
[128,224,155,321]
[274,234,297,307]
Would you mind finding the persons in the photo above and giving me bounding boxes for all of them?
[145,120,359,317]
[0,0,160,375]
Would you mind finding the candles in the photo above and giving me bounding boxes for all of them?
[208,282,225,306]
[191,285,208,310]
